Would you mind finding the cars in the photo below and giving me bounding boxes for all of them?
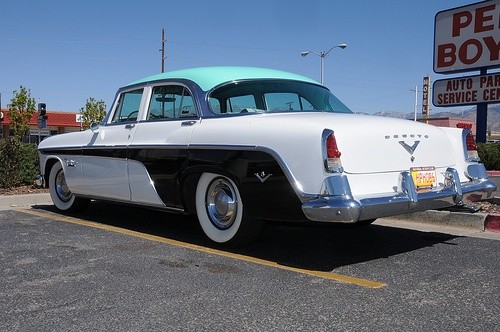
[37,65,498,249]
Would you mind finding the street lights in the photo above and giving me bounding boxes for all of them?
[301,43,347,84]
